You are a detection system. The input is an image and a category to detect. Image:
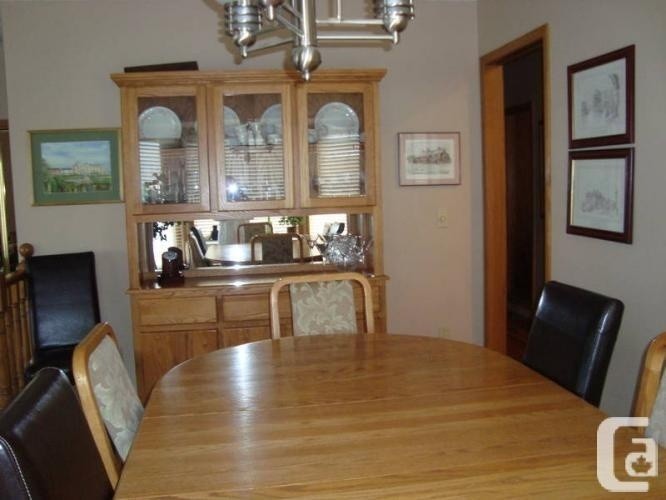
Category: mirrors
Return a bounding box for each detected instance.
[136,213,374,280]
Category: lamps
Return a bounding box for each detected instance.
[221,1,416,83]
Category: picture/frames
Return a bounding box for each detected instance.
[565,147,634,244]
[26,128,125,207]
[567,44,635,149]
[398,131,461,186]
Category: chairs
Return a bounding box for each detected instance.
[0,366,115,500]
[629,331,666,436]
[72,322,145,491]
[189,223,345,266]
[269,272,375,339]
[24,252,100,385]
[522,280,625,408]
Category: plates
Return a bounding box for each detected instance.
[139,101,360,205]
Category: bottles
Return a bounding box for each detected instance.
[211,225,219,240]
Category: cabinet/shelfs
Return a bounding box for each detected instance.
[110,68,389,409]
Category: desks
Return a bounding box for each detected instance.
[111,333,666,500]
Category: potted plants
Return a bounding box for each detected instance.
[279,216,305,235]
